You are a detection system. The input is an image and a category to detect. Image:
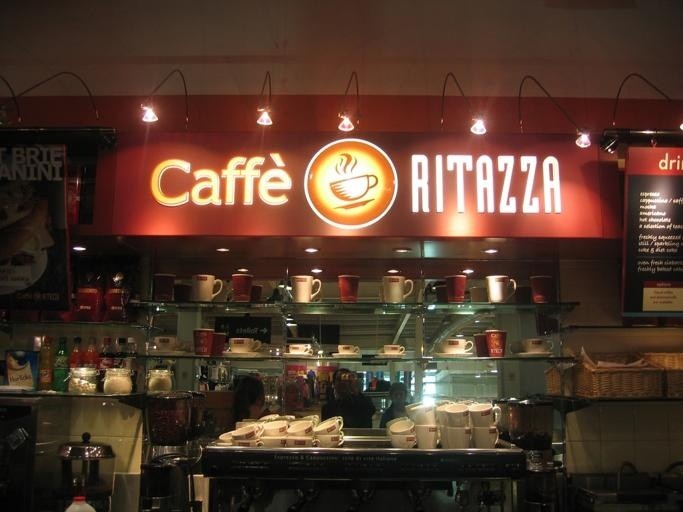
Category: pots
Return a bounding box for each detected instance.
[493,392,555,450]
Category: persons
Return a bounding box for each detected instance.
[322,368,374,434]
[222,371,269,431]
[378,383,419,431]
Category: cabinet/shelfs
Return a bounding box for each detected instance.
[0,302,578,476]
[204,437,524,512]
[562,327,683,512]
[1,390,143,511]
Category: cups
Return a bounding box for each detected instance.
[484,329,506,357]
[383,345,404,354]
[337,274,359,302]
[444,275,467,303]
[286,420,316,436]
[391,434,417,449]
[231,274,253,302]
[409,402,439,424]
[522,338,553,352]
[216,442,233,446]
[258,436,286,447]
[213,332,226,355]
[232,424,264,439]
[386,399,480,442]
[153,337,176,351]
[288,344,311,353]
[445,426,473,449]
[257,413,342,428]
[389,420,416,435]
[485,275,516,303]
[529,275,556,303]
[439,338,474,352]
[382,275,413,304]
[228,338,260,353]
[290,275,321,303]
[337,345,359,354]
[472,333,488,357]
[285,436,320,448]
[261,420,288,436]
[192,328,214,355]
[230,438,264,447]
[190,275,223,301]
[439,424,449,448]
[312,418,343,435]
[436,404,451,426]
[445,403,471,427]
[314,434,344,448]
[218,429,236,443]
[414,425,440,448]
[471,426,499,448]
[468,403,502,427]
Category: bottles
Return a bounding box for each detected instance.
[54,338,69,393]
[126,338,137,394]
[65,497,95,512]
[115,336,126,369]
[83,337,98,369]
[70,337,82,372]
[97,337,113,393]
[38,336,53,391]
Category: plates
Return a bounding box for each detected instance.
[331,353,362,357]
[375,353,406,359]
[429,352,474,358]
[282,352,312,358]
[518,351,554,357]
[222,351,260,358]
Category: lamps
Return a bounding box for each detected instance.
[518,75,591,148]
[140,67,191,131]
[256,71,275,128]
[3,71,101,126]
[337,71,363,132]
[440,72,486,135]
[612,73,683,131]
[599,128,629,155]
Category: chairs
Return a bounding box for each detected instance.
[138,393,204,512]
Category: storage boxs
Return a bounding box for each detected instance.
[644,281,683,311]
[638,352,681,397]
[574,354,666,398]
[544,364,573,395]
